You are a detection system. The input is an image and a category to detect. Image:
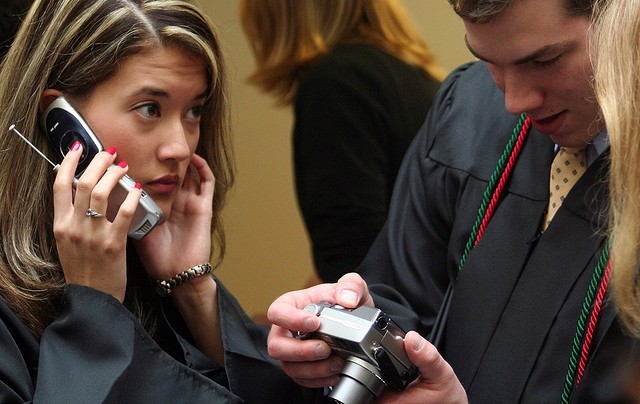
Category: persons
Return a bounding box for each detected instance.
[236,0,452,285]
[266,1,640,404]
[583,0,640,341]
[0,0,333,404]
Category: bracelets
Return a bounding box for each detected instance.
[153,261,213,298]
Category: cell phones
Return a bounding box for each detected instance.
[7,96,164,242]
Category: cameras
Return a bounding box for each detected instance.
[297,300,422,404]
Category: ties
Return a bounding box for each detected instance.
[543,146,588,234]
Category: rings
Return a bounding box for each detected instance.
[85,207,106,218]
